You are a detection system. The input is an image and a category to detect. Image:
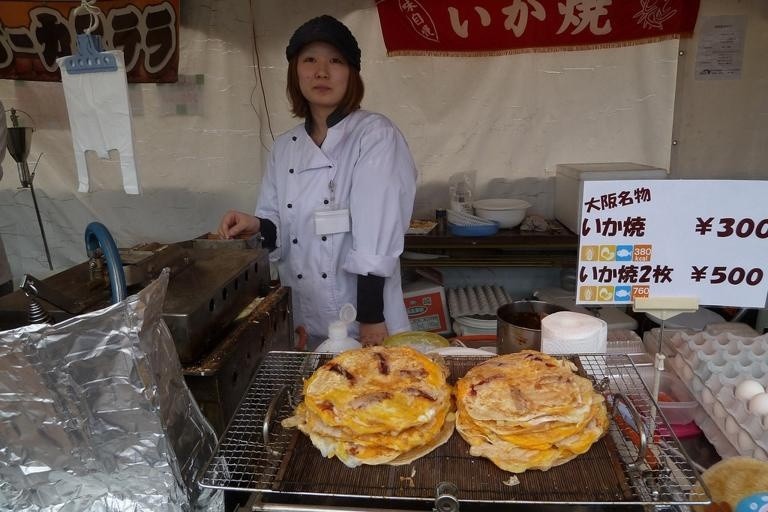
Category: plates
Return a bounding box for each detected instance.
[445,208,501,236]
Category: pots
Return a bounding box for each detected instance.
[494,302,572,357]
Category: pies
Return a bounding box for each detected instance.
[296,345,455,473]
[453,349,609,473]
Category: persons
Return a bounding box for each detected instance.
[217,14,419,347]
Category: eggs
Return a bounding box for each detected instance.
[674,353,768,462]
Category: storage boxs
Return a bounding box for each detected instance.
[542,297,638,330]
[632,371,699,425]
[553,163,668,235]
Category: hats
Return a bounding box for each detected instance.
[286,15,361,67]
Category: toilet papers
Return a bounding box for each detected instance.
[541,309,607,380]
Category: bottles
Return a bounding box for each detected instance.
[312,304,361,354]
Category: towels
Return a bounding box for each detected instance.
[520,215,549,232]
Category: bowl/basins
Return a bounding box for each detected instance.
[472,198,532,229]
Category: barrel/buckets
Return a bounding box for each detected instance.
[450,312,497,354]
